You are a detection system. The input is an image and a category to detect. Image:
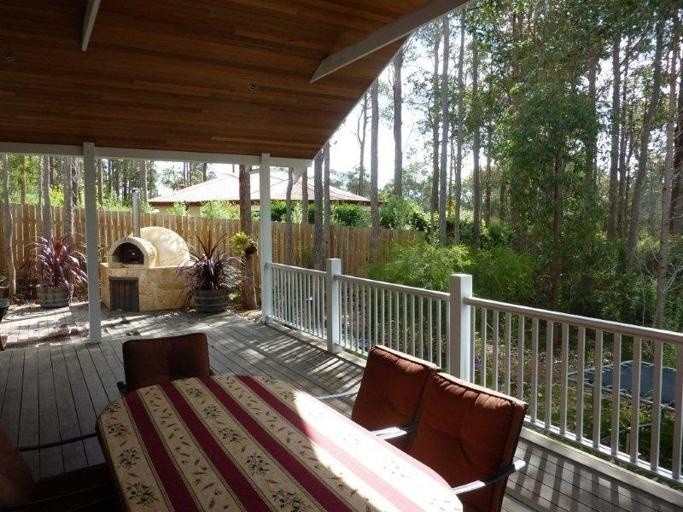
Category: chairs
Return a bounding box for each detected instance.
[377,371,529,511]
[1,419,128,512]
[315,344,442,453]
[116,333,216,395]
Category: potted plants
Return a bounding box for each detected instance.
[21,232,88,308]
[175,234,243,313]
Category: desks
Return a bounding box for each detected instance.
[94,374,462,512]
